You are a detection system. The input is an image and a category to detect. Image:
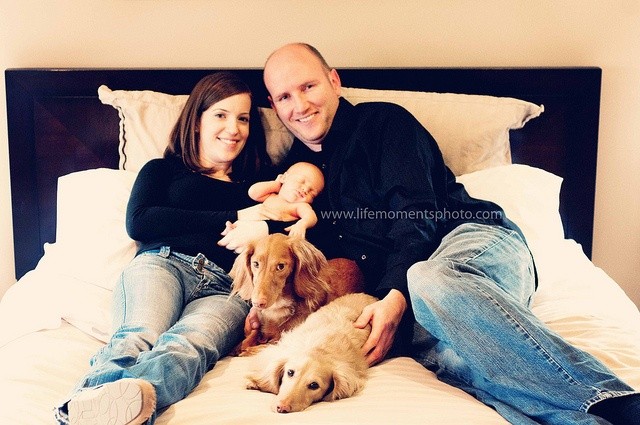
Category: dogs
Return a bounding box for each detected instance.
[227,233,365,356]
[242,293,380,414]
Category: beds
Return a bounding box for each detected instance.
[3,65,640,424]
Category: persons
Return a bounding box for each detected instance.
[226,162,325,239]
[51,70,340,425]
[244,43,639,425]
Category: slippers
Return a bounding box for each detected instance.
[68,379,158,425]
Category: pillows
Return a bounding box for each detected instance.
[344,86,546,174]
[97,83,294,171]
